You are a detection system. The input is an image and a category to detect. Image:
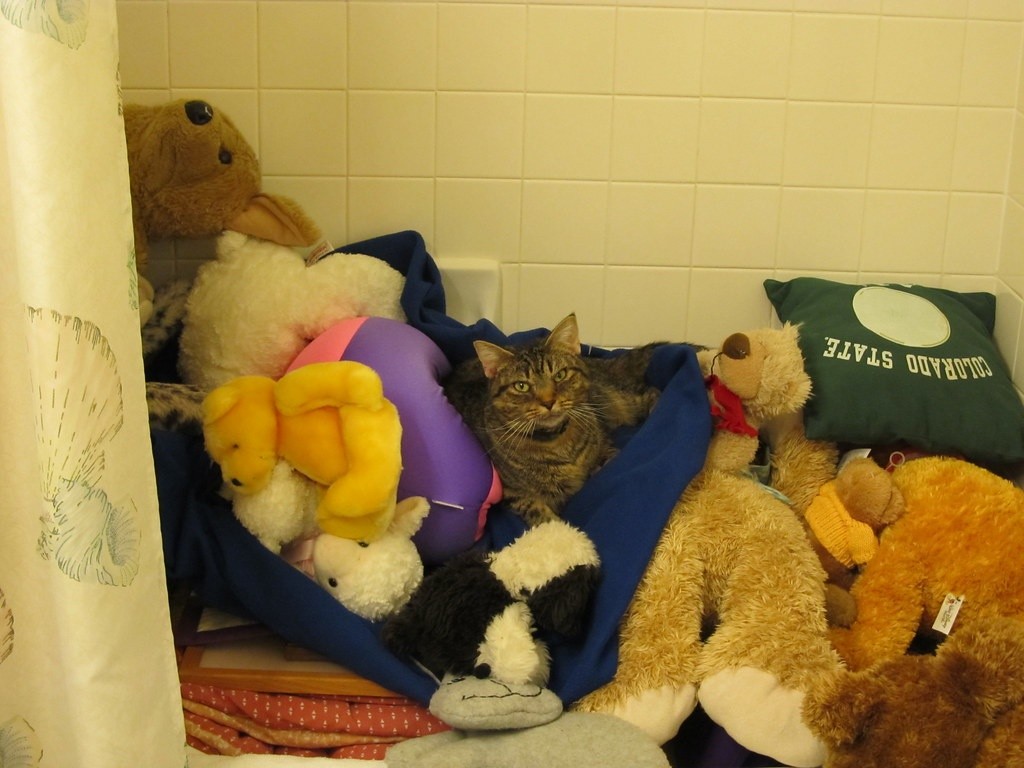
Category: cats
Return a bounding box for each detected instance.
[450,312,710,526]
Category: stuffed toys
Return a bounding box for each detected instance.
[121,96,1024,768]
